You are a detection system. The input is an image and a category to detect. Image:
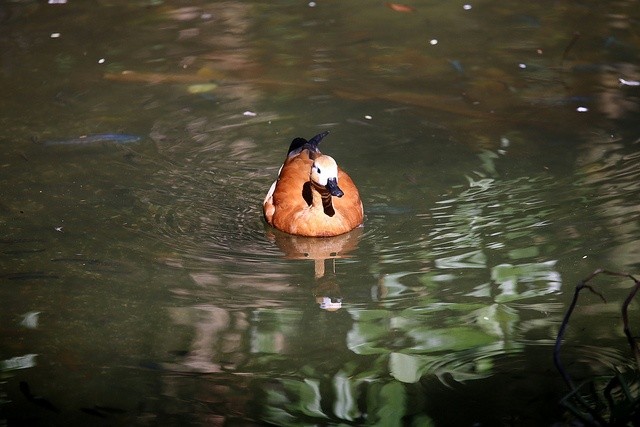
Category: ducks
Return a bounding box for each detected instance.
[263,129,365,237]
